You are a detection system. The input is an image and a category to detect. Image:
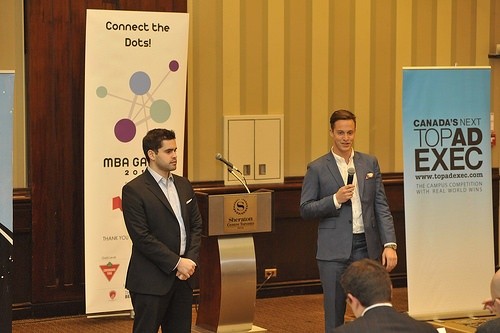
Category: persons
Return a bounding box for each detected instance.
[475,268,500,333]
[299,109,397,333]
[334,258,439,333]
[122,129,202,333]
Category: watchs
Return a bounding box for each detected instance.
[384,244,397,250]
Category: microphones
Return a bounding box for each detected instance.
[347,167,356,184]
[216,153,241,173]
